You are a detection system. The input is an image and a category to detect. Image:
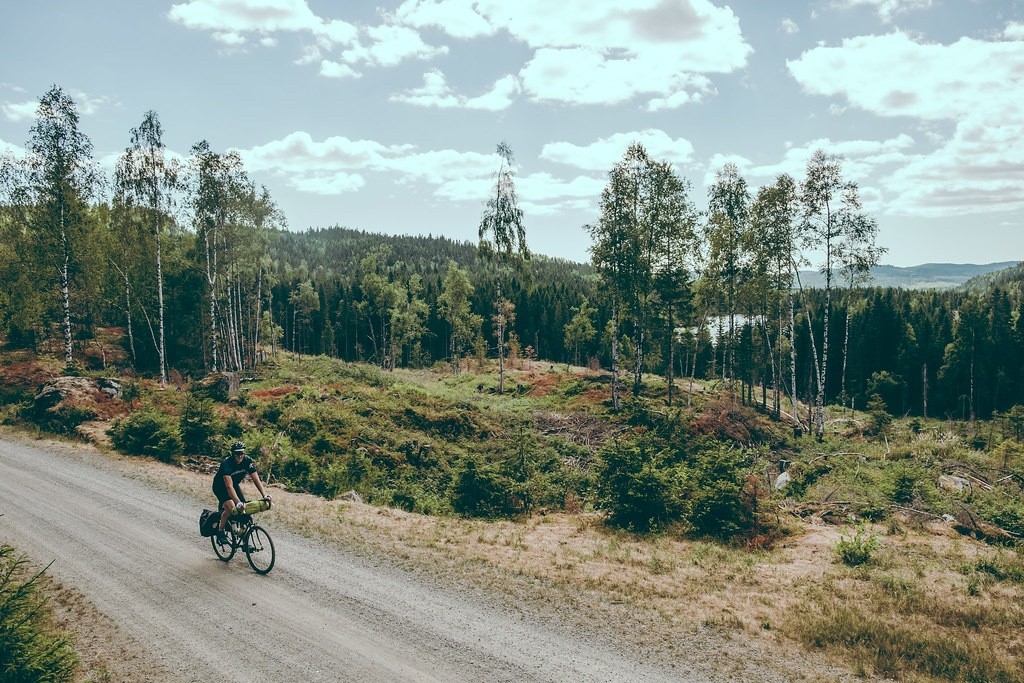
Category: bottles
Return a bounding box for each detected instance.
[239,524,246,537]
[231,522,237,533]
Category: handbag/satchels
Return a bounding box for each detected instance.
[200,508,221,538]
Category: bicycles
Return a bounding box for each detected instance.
[210,495,275,574]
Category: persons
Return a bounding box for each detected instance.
[212,443,272,552]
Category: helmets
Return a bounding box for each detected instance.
[231,441,246,452]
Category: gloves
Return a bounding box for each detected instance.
[235,501,246,513]
[264,495,272,500]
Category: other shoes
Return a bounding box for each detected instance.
[242,545,253,552]
[217,531,228,543]
